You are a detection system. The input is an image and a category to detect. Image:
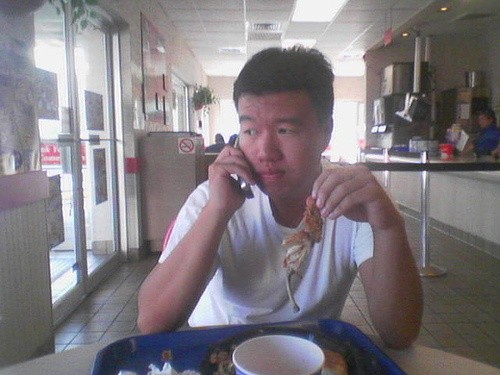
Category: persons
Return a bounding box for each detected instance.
[468,107,499,158]
[138,43,424,349]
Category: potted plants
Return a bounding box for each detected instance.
[195,82,219,111]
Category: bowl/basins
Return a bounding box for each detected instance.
[232,335,325,375]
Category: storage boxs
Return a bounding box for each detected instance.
[372,96,406,127]
[371,121,428,153]
[381,60,429,95]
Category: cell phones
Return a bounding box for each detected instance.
[233,135,255,199]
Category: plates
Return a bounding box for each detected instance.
[203,326,386,375]
[92,320,404,374]
